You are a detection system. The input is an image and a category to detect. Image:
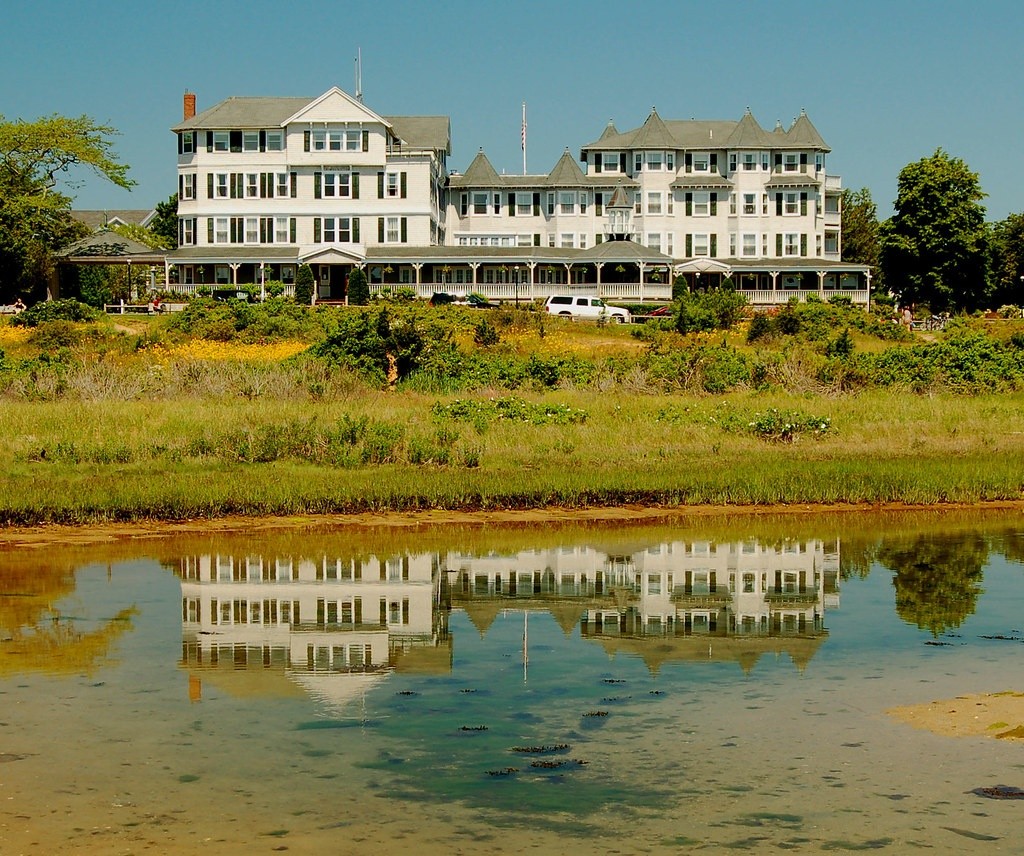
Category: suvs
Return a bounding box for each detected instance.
[544,295,631,324]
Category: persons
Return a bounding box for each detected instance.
[13,298,27,315]
[904,306,913,331]
[152,296,163,315]
